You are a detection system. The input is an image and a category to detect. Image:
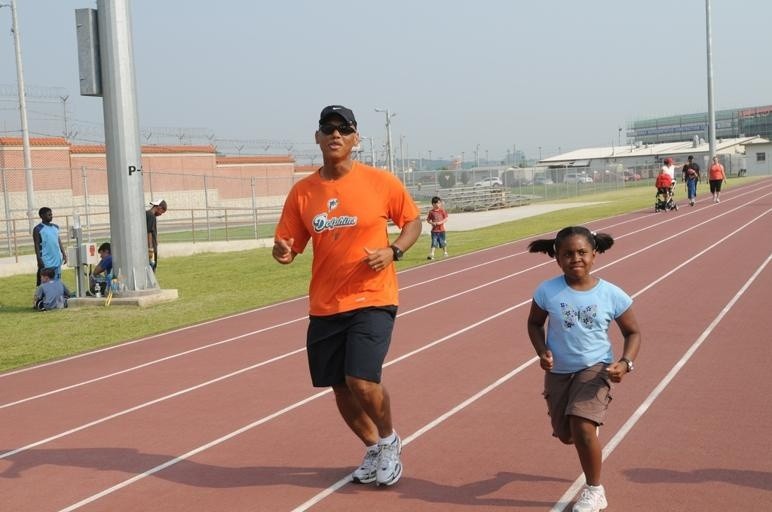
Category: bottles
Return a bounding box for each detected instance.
[95,282,101,297]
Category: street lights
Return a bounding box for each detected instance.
[360,135,375,168]
[426,144,562,166]
[618,127,623,145]
[375,108,399,176]
[399,134,406,160]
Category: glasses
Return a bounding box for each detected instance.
[322,125,354,136]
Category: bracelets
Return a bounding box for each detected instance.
[619,357,633,373]
[390,244,403,261]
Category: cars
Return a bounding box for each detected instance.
[475,177,503,190]
[563,173,593,185]
[587,170,640,181]
[527,176,553,186]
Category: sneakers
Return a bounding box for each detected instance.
[353,434,402,487]
[572,484,608,512]
[427,255,435,259]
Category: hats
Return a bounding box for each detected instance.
[150,200,167,211]
[320,106,357,132]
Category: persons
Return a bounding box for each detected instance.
[85,243,113,296]
[686,168,697,207]
[33,207,69,286]
[32,268,70,311]
[706,156,727,204]
[526,225,643,511]
[146,199,168,273]
[273,105,423,488]
[426,196,449,260]
[658,156,675,204]
[681,154,700,196]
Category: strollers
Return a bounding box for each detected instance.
[655,174,679,214]
